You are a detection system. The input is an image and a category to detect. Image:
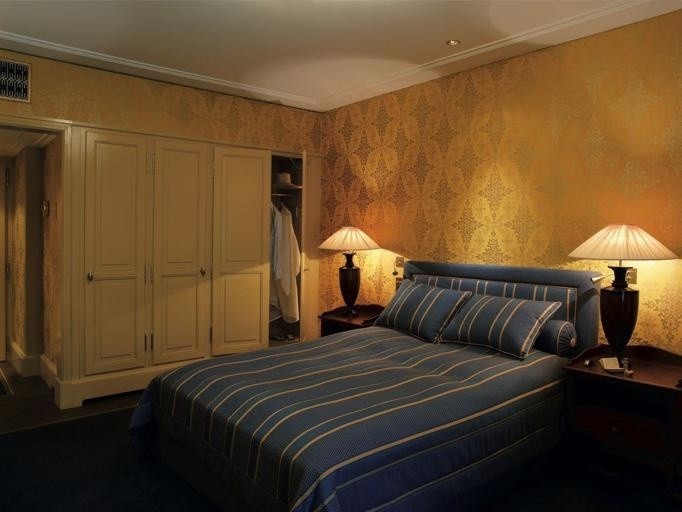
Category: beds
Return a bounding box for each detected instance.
[128,260,602,509]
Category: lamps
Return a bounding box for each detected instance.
[317,226,380,319]
[568,224,680,377]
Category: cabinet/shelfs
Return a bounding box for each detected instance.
[60,124,322,411]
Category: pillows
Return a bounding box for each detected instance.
[373,278,578,362]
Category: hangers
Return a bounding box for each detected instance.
[271,196,291,217]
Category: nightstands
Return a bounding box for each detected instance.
[318,303,386,337]
[563,343,680,511]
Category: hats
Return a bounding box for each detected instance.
[272,172,302,189]
